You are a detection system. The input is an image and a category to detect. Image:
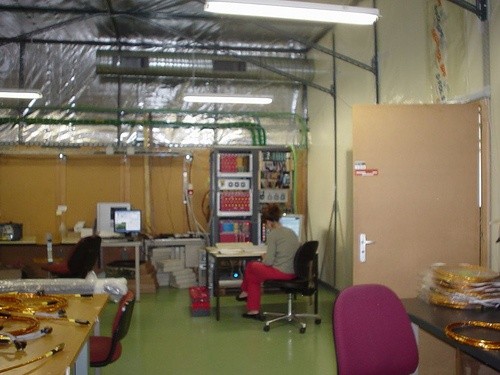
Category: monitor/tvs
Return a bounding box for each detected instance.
[114,209,142,239]
[280,214,304,241]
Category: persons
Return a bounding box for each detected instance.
[236,205,301,320]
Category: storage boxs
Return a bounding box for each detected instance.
[107,246,200,293]
[0,268,22,281]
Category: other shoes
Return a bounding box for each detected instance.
[236,294,247,301]
[241,312,262,320]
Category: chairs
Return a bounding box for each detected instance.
[41,234,102,279]
[332,284,419,375]
[88,290,135,368]
[260,240,322,333]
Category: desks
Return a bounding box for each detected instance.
[400,297,500,375]
[0,275,128,375]
[203,247,318,320]
[0,237,206,278]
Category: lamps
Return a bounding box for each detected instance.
[183,80,273,105]
[203,0,380,26]
[0,88,44,99]
